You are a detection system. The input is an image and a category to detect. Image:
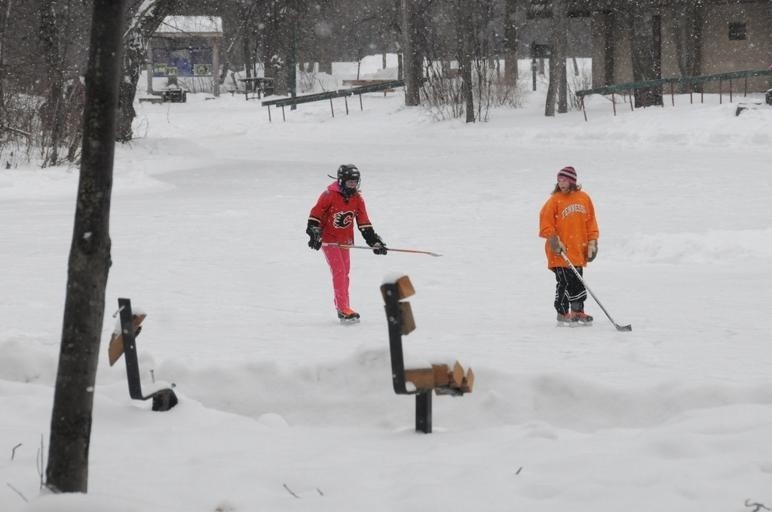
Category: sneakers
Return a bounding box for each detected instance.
[556,311,593,323]
[337,312,361,319]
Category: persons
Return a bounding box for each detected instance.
[306,164,388,320]
[538,167,599,322]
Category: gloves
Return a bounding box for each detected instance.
[366,233,387,255]
[305,225,322,251]
[549,235,566,256]
[586,239,598,262]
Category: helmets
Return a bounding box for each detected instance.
[338,164,361,193]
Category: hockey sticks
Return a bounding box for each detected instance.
[308,241,442,258]
[559,249,632,331]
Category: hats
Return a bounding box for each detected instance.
[557,166,577,184]
